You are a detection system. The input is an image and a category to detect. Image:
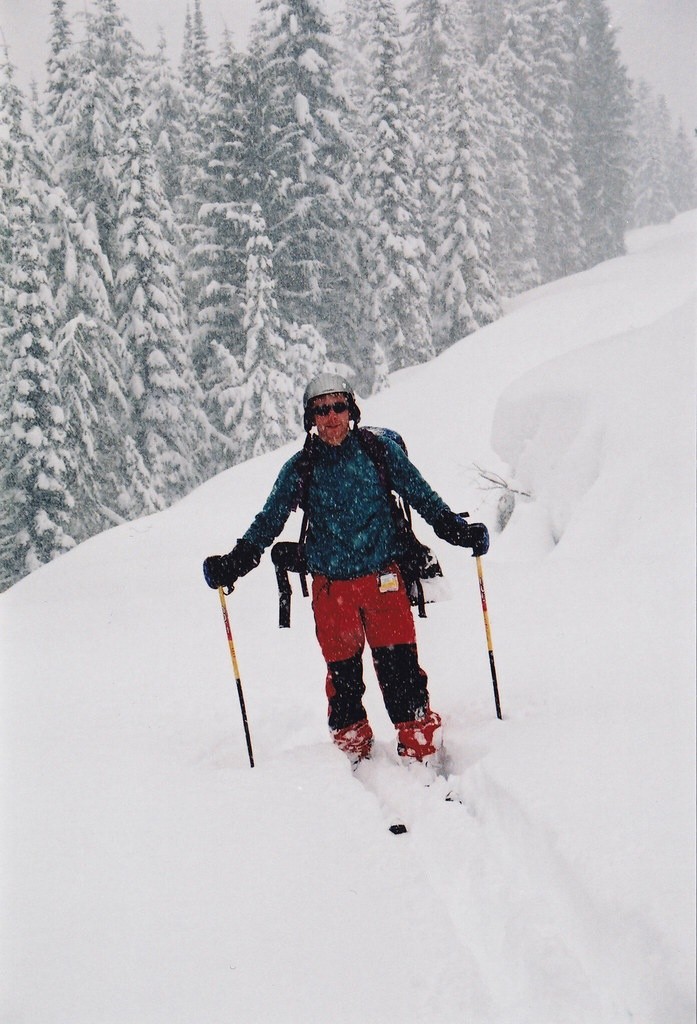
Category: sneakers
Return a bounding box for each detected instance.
[395,712,441,766]
[328,719,374,773]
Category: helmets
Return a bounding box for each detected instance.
[303,372,355,411]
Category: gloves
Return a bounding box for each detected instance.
[433,510,490,557]
[203,539,264,595]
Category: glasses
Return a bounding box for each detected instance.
[311,401,349,416]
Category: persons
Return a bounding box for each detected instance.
[202,374,490,777]
